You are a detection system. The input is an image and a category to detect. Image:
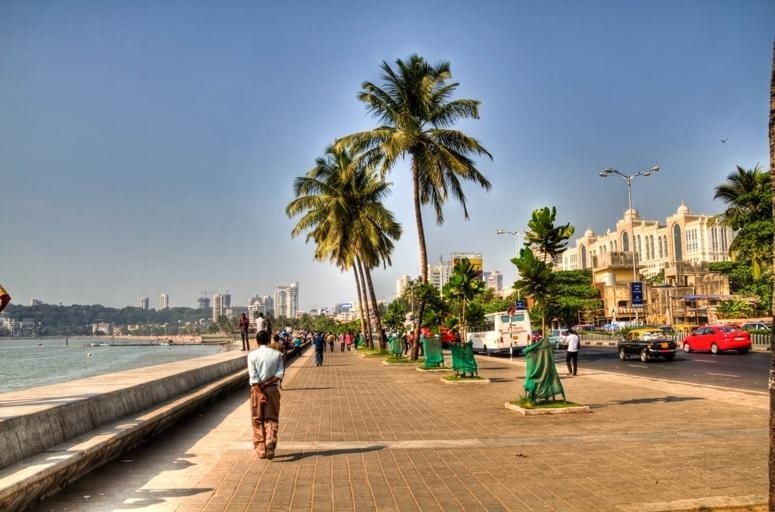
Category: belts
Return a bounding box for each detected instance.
[267,384,278,386]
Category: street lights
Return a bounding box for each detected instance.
[598,166,660,329]
[496,228,527,310]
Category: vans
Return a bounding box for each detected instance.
[548,328,570,349]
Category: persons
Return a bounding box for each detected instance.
[247,330,286,460]
[266,317,371,390]
[239,312,250,351]
[254,312,265,333]
[562,328,580,376]
[382,329,425,358]
[531,327,542,342]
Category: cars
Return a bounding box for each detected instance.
[657,326,676,333]
[573,324,596,332]
[532,329,543,343]
[741,322,770,331]
[618,329,677,363]
[682,324,752,356]
[600,323,620,334]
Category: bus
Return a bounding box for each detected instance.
[465,309,533,357]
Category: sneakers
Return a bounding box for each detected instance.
[266,441,275,460]
